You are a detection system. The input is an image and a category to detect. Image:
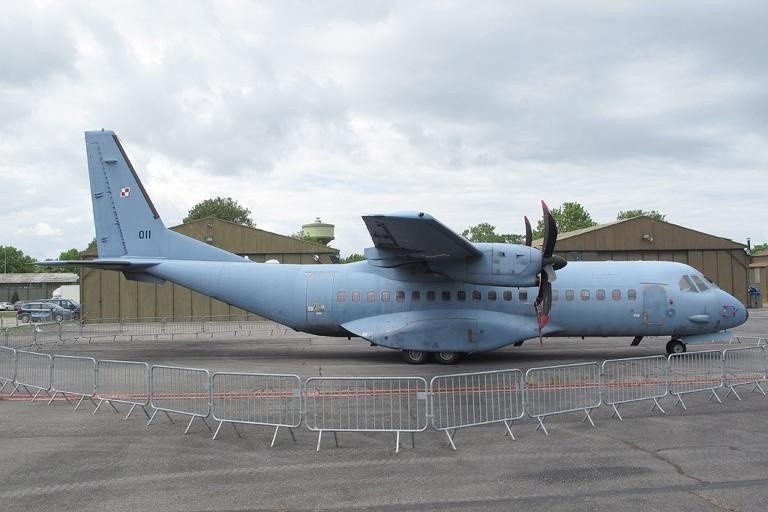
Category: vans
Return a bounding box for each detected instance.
[44,299,79,315]
[16,302,74,323]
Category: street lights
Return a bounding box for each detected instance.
[2,243,7,274]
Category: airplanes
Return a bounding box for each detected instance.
[25,129,749,365]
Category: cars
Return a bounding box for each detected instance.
[0,302,14,311]
[14,300,23,310]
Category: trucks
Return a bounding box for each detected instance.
[49,285,80,306]
